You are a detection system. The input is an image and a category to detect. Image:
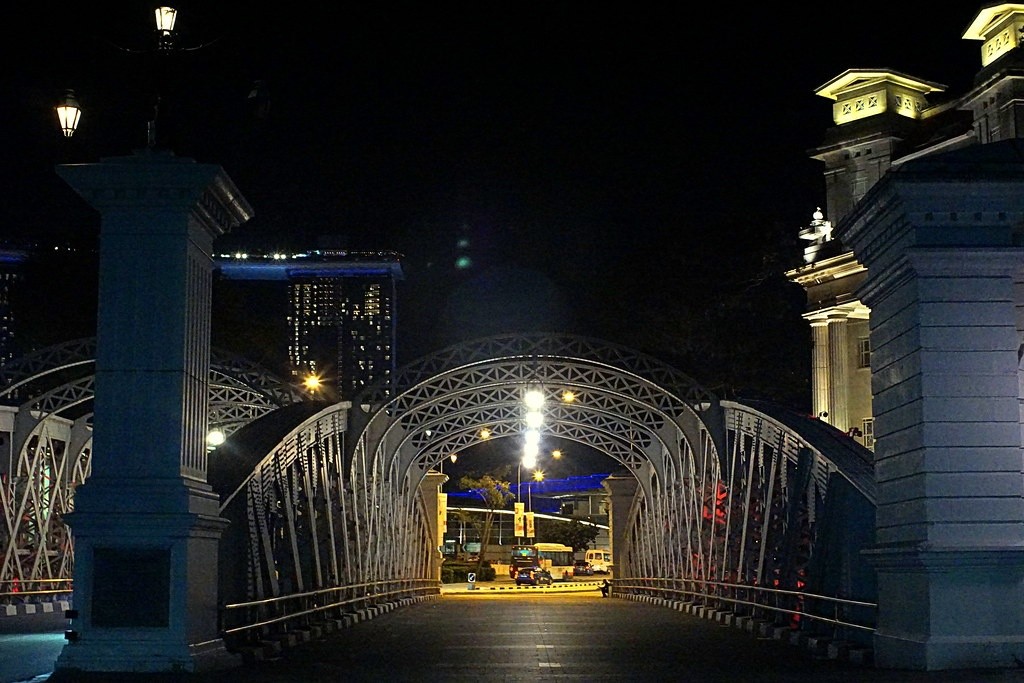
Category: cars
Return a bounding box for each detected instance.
[574,562,595,575]
[515,567,558,584]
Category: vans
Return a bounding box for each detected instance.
[586,549,612,574]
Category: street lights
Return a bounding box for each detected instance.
[528,470,545,544]
[517,450,561,544]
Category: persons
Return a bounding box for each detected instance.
[599,579,610,598]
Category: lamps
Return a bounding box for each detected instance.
[846,427,862,438]
[49,89,82,154]
[819,411,829,418]
[153,0,178,38]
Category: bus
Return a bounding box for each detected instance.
[509,543,574,581]
